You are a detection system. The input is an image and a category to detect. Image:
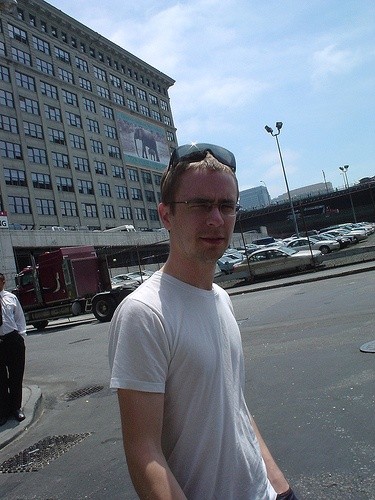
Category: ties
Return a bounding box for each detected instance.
[0,300,3,327]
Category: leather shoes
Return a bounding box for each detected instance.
[0,412,8,425]
[14,408,25,422]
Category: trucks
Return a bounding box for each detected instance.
[11,245,112,330]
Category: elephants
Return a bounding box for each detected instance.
[133,126,161,162]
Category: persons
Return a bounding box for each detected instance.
[109,143,296,500]
[0,272,27,426]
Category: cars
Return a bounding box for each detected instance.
[230,246,324,282]
[286,236,341,254]
[215,235,282,276]
[91,270,155,323]
[283,222,375,250]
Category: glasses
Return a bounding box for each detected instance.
[161,143,236,189]
[164,200,247,216]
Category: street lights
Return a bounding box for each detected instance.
[265,121,299,237]
[338,164,357,223]
